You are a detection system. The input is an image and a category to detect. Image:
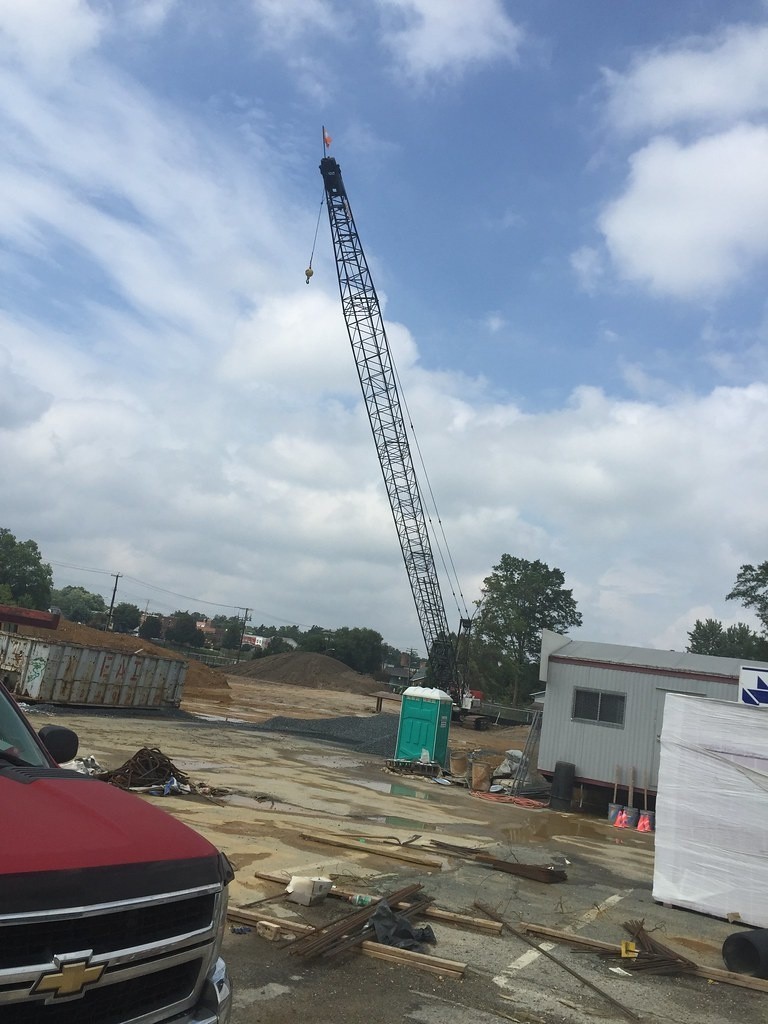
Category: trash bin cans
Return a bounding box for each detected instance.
[394,693,454,769]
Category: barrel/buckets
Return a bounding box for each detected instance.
[450,751,468,775]
[608,803,623,824]
[623,806,638,826]
[472,760,490,791]
[640,810,655,828]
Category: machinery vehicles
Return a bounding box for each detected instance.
[305,126,491,731]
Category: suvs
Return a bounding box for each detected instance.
[0,678,233,1024]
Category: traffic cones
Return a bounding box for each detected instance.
[635,814,644,831]
[642,814,651,831]
[619,811,629,828]
[614,809,622,826]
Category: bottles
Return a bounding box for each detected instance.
[349,894,371,906]
